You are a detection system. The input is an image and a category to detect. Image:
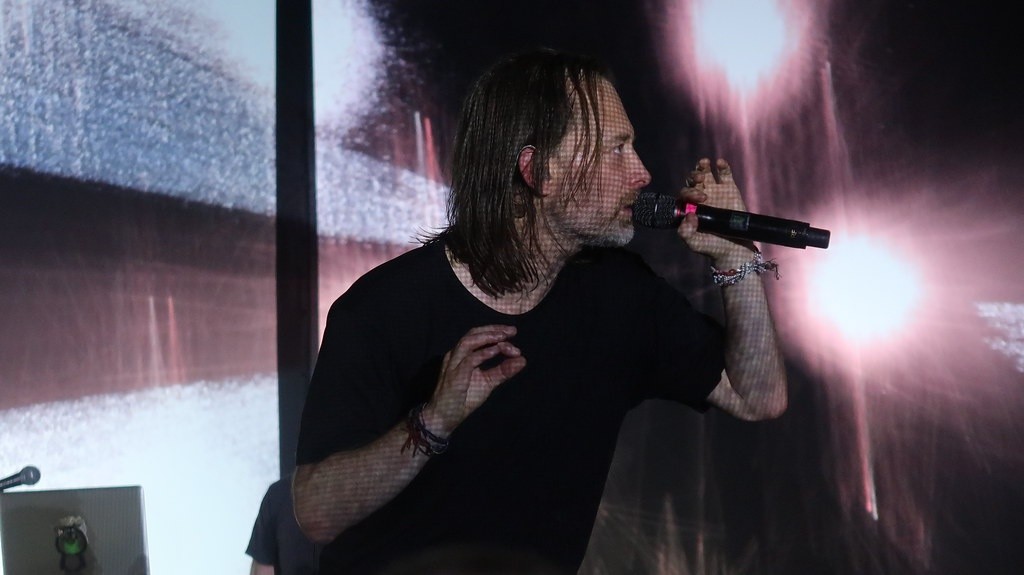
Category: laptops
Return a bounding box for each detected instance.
[0,486,150,575]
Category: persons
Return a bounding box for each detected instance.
[246,468,325,575]
[293,47,790,575]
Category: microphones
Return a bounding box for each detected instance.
[632,192,831,250]
[0,466,41,489]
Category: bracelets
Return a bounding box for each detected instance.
[710,250,780,287]
[402,404,449,459]
